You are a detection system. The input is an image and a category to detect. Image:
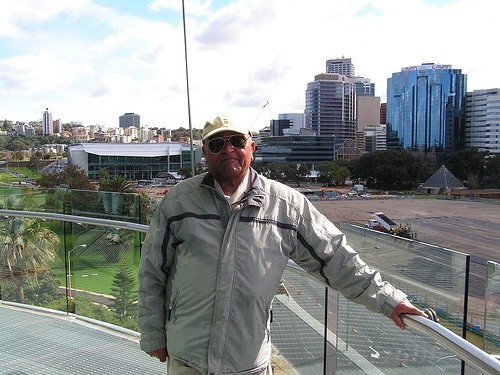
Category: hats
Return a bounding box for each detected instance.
[201,113,253,141]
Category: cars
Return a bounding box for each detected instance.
[306,185,400,201]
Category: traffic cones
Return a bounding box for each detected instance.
[315,297,320,305]
[398,356,404,367]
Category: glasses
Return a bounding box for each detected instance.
[204,135,250,153]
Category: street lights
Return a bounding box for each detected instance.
[67,243,87,301]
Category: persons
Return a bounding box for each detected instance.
[138,113,429,375]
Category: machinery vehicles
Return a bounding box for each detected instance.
[364,211,397,235]
